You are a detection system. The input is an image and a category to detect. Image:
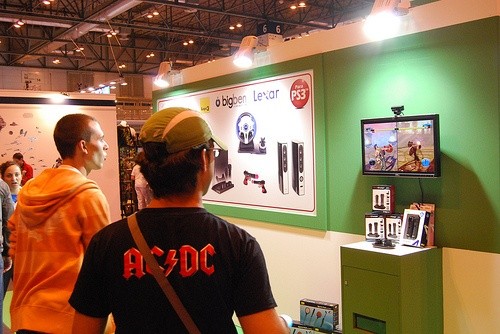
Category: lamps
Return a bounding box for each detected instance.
[152,62,171,89]
[369,0,411,16]
[232,36,258,68]
[78,80,127,94]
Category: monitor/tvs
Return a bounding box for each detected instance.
[361,113,441,179]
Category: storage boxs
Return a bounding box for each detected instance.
[289,298,341,334]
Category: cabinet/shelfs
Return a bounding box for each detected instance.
[341,241,443,334]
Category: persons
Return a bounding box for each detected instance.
[131,152,151,210]
[0,160,24,211]
[67,107,292,334]
[6,113,116,334]
[12,153,33,186]
[0,113,14,333]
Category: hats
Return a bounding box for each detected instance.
[139,108,227,167]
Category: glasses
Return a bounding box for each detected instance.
[208,148,220,158]
